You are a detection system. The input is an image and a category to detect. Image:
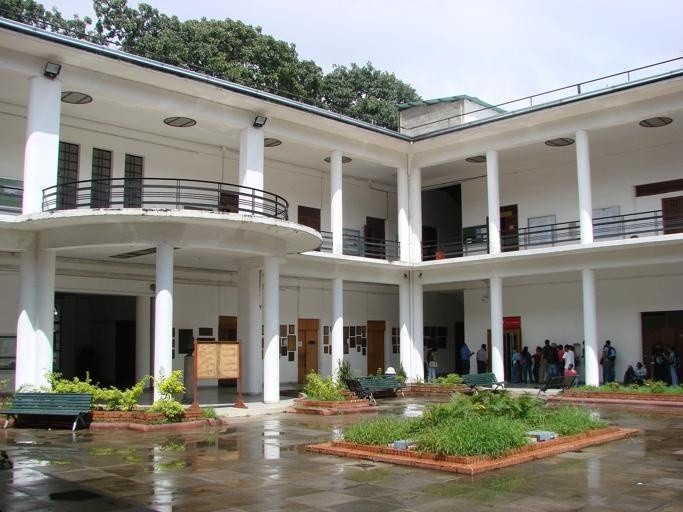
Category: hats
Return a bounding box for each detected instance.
[385,367,396,374]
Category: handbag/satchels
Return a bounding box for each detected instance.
[429,360,438,367]
[607,347,616,360]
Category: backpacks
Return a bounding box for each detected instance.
[672,356,681,368]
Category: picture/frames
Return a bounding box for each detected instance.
[280,325,297,360]
[391,327,448,353]
[324,326,366,355]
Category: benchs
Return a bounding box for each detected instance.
[0,392,93,432]
[346,375,405,405]
[534,376,577,397]
[462,372,504,395]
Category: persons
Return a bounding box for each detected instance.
[601,340,616,384]
[512,339,578,387]
[461,344,475,374]
[624,340,683,389]
[581,341,584,358]
[424,343,439,384]
[477,344,488,373]
